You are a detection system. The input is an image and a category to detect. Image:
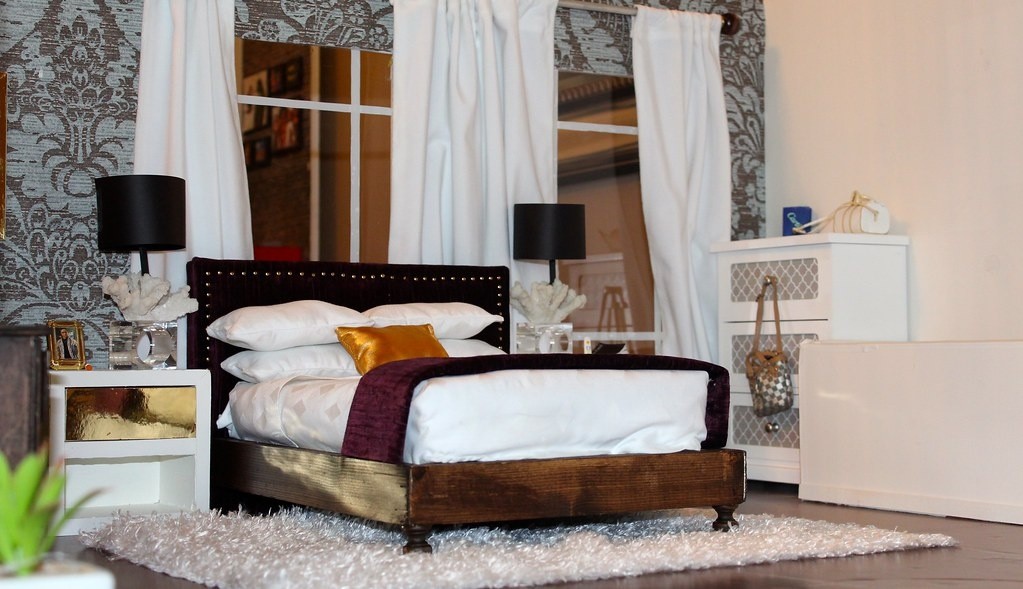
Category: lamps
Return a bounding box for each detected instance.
[512,203,585,284]
[95,175,186,275]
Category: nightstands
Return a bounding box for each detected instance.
[47,369,211,536]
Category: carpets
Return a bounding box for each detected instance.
[76,505,955,589]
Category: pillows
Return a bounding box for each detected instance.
[206,299,375,353]
[440,338,506,358]
[221,343,362,384]
[362,303,504,339]
[336,323,450,377]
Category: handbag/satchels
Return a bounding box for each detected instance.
[746,349,794,417]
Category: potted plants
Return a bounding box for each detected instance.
[0,441,115,588]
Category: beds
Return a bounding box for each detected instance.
[186,257,747,555]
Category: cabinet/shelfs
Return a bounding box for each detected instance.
[710,232,911,483]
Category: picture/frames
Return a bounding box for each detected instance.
[49,320,86,370]
[242,56,304,170]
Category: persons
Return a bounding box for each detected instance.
[56,329,79,359]
